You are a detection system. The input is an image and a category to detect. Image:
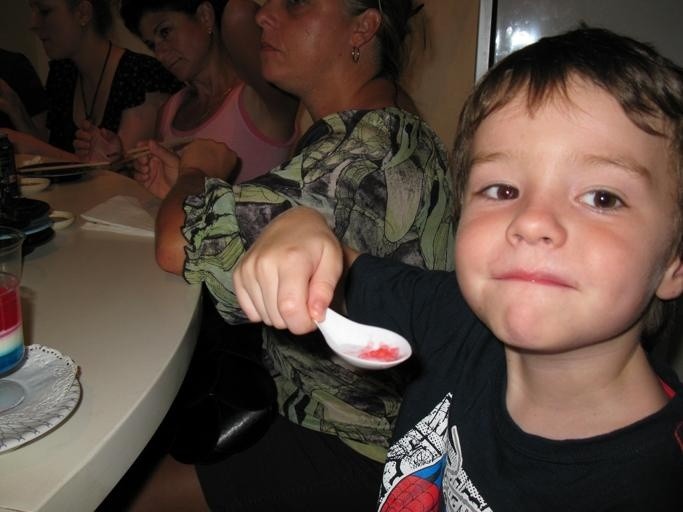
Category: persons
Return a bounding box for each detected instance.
[120,2,459,511]
[72,0,304,189]
[231,22,682,511]
[0,48,48,145]
[0,0,186,164]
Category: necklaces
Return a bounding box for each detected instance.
[175,75,244,131]
[71,37,115,125]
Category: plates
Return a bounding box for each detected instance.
[48,211,73,230]
[0,345,82,454]
[18,177,49,194]
[19,162,92,183]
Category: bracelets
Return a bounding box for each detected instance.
[174,166,212,186]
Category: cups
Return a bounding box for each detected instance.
[0,222,29,376]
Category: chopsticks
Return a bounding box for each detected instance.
[126,138,189,162]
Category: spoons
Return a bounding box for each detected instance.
[312,296,413,376]
